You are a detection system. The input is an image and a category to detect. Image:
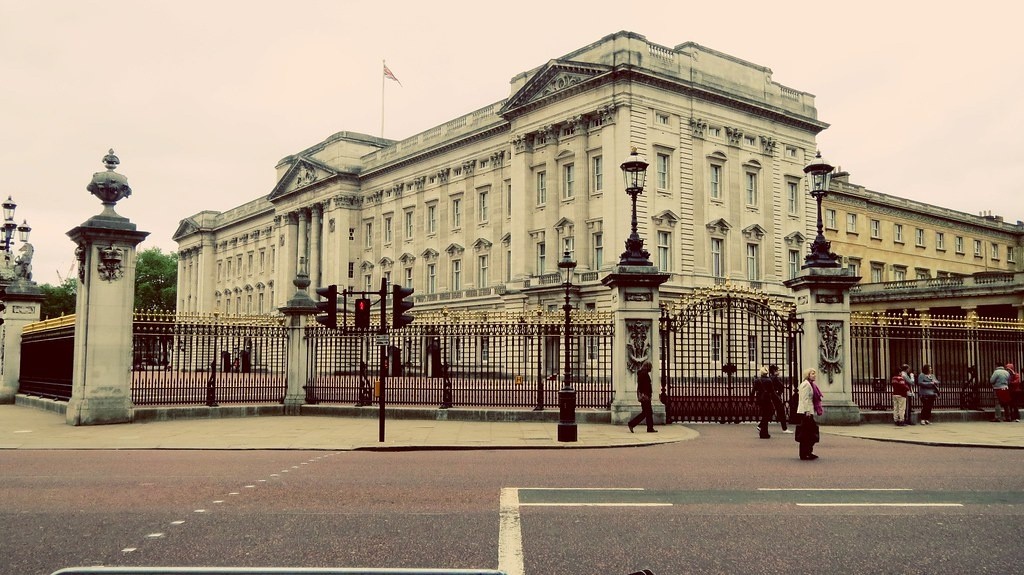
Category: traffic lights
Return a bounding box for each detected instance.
[393,284,415,330]
[315,284,338,329]
[354,299,370,330]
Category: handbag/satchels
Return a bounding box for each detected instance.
[795,416,820,442]
[638,392,650,402]
[934,384,941,396]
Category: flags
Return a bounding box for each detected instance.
[383,65,402,87]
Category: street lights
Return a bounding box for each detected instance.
[802,148,844,267]
[0,195,17,253]
[617,145,659,266]
[557,246,578,441]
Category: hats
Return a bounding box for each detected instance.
[760,367,769,376]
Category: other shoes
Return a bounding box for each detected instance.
[783,429,794,433]
[628,421,635,433]
[921,419,930,425]
[906,420,916,425]
[1013,418,1020,421]
[760,435,770,439]
[800,454,818,460]
[990,417,1000,422]
[647,428,658,432]
[757,426,761,432]
[895,421,906,426]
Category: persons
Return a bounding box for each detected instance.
[1005,364,1021,422]
[891,367,910,427]
[918,365,939,426]
[900,364,916,425]
[796,369,819,461]
[990,363,1011,422]
[749,367,778,439]
[628,360,658,433]
[757,365,793,434]
